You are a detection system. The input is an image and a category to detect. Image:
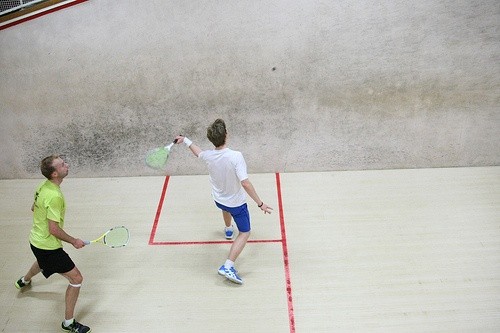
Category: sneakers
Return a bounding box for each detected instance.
[15,276,31,289]
[218,265,243,284]
[61,318,94,333]
[224,224,233,238]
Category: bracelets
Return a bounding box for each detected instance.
[257,202,263,207]
[183,137,192,148]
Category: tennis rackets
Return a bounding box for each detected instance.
[144,135,182,169]
[82,224,130,249]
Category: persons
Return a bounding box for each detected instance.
[174,119,273,285]
[15,155,92,333]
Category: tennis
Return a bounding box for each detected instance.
[271,65,277,72]
